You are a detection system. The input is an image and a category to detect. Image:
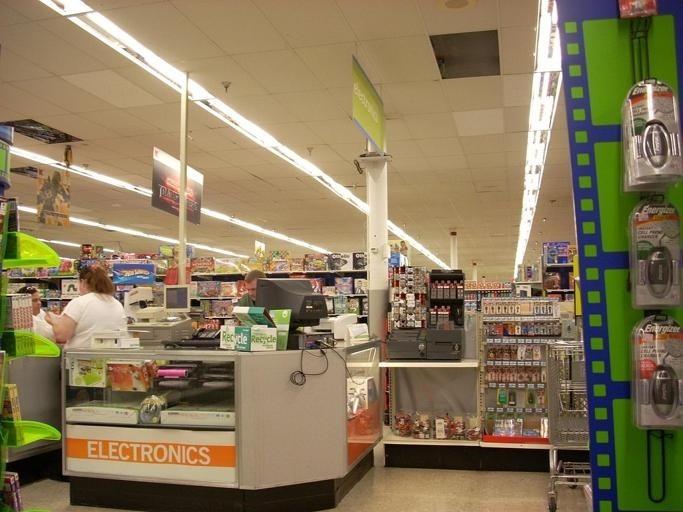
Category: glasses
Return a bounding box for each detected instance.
[24,286,37,293]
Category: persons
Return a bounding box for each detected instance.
[235,268,267,307]
[37,169,69,226]
[43,264,128,349]
[15,286,57,343]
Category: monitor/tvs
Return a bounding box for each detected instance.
[256,279,328,332]
[163,285,191,312]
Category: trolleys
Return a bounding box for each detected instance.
[539,341,595,512]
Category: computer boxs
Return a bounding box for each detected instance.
[288,332,334,350]
